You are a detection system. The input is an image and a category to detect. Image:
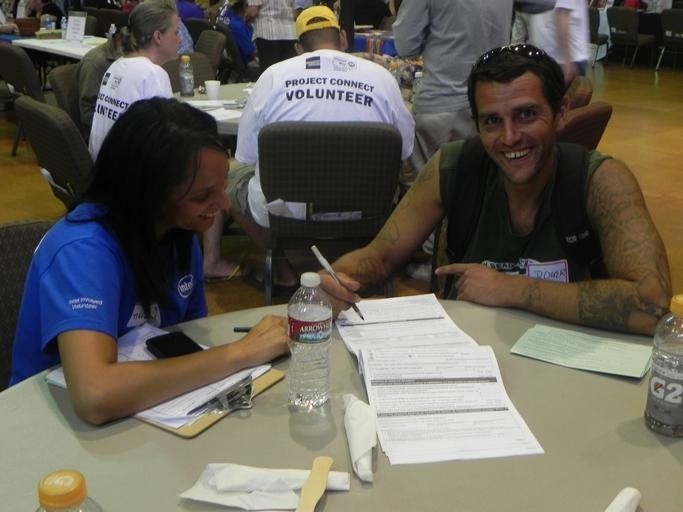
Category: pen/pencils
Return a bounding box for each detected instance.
[234,327,252,336]
[311,245,367,320]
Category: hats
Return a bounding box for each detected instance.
[294,5,340,38]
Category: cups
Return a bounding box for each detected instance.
[204,79,221,101]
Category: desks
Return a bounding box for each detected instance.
[0,293,680,510]
[170,79,422,139]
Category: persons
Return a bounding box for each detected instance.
[11,96,301,425]
[168,1,297,75]
[294,44,671,341]
[211,5,420,297]
[0,1,137,143]
[389,0,559,284]
[555,0,591,85]
[80,0,255,281]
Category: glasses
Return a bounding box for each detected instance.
[474,43,545,69]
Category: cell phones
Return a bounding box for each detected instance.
[146,331,203,359]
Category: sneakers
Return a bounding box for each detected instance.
[256,263,299,295]
[407,260,431,282]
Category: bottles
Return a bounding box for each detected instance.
[44,14,49,30]
[178,56,195,98]
[108,24,116,34]
[32,470,106,512]
[287,271,332,406]
[60,17,67,40]
[412,72,425,100]
[642,292,682,437]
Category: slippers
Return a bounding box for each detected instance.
[205,264,248,283]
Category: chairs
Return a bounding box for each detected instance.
[586,6,681,72]
[553,99,612,153]
[12,95,97,209]
[8,2,402,95]
[47,62,81,139]
[561,75,592,112]
[250,115,408,310]
[0,42,48,156]
